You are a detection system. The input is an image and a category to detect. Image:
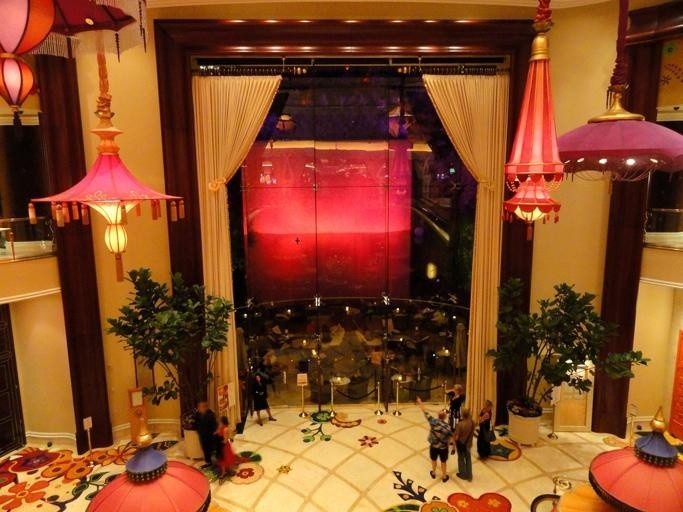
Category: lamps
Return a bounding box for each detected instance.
[500,0,566,242]
[0,1,148,144]
[386,102,414,139]
[23,19,189,285]
[555,2,682,185]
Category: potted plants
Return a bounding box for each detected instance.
[102,268,235,460]
[481,282,653,446]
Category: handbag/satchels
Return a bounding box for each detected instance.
[489,430,496,442]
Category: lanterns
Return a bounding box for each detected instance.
[2,1,54,143]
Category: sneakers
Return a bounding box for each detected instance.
[430,471,435,478]
[443,475,449,482]
[456,473,466,480]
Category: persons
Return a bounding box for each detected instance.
[214,415,238,479]
[251,372,277,426]
[192,395,224,468]
[416,384,492,482]
